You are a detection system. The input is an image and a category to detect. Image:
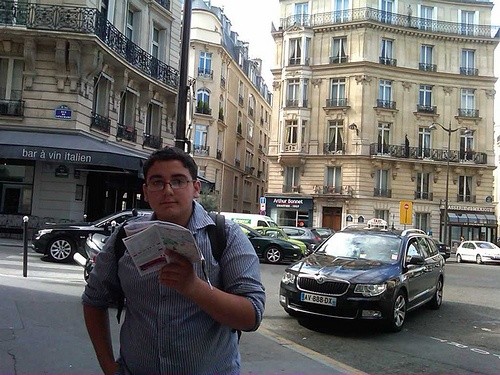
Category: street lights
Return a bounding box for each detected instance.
[428,121,472,244]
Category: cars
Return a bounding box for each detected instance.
[455,240,500,265]
[278,219,445,333]
[30,210,155,264]
[430,237,451,262]
[238,223,303,264]
[247,223,336,258]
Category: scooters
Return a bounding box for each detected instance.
[83,207,138,284]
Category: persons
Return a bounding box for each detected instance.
[460,236,465,242]
[297,221,308,227]
[82,146,266,375]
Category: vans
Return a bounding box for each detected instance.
[211,210,280,235]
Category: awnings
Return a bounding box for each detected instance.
[446,212,497,224]
[0,130,149,170]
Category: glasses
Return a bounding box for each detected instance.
[148,179,197,192]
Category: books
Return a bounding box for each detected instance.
[122,221,203,277]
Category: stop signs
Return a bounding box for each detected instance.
[404,204,409,210]
